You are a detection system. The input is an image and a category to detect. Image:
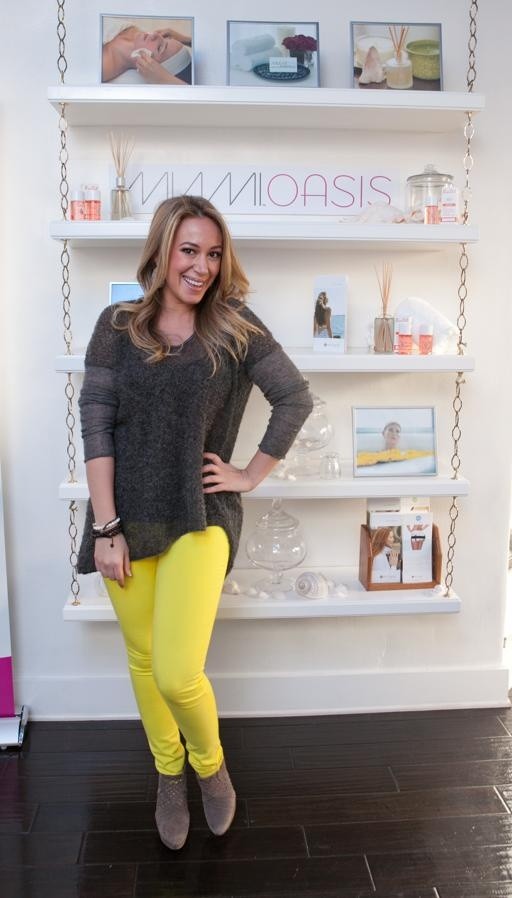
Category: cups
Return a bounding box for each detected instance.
[318,451,343,480]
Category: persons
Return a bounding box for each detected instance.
[372,527,400,583]
[358,421,434,466]
[102,16,190,83]
[75,195,312,850]
[136,26,192,85]
[314,290,333,338]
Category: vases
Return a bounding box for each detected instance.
[290,50,313,67]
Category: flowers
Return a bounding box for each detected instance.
[282,33,317,53]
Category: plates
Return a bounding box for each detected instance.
[404,39,441,57]
[252,62,312,81]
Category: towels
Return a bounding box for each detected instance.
[230,34,283,72]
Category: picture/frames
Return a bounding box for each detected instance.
[349,21,444,92]
[108,281,142,307]
[226,19,321,88]
[350,404,440,477]
[99,13,196,89]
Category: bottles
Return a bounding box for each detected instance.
[404,162,455,225]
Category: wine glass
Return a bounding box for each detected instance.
[245,499,309,595]
[276,381,334,480]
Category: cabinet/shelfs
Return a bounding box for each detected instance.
[43,80,487,620]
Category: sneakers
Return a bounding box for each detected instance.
[195,759,238,837]
[154,764,190,851]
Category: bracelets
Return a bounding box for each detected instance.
[89,516,124,549]
